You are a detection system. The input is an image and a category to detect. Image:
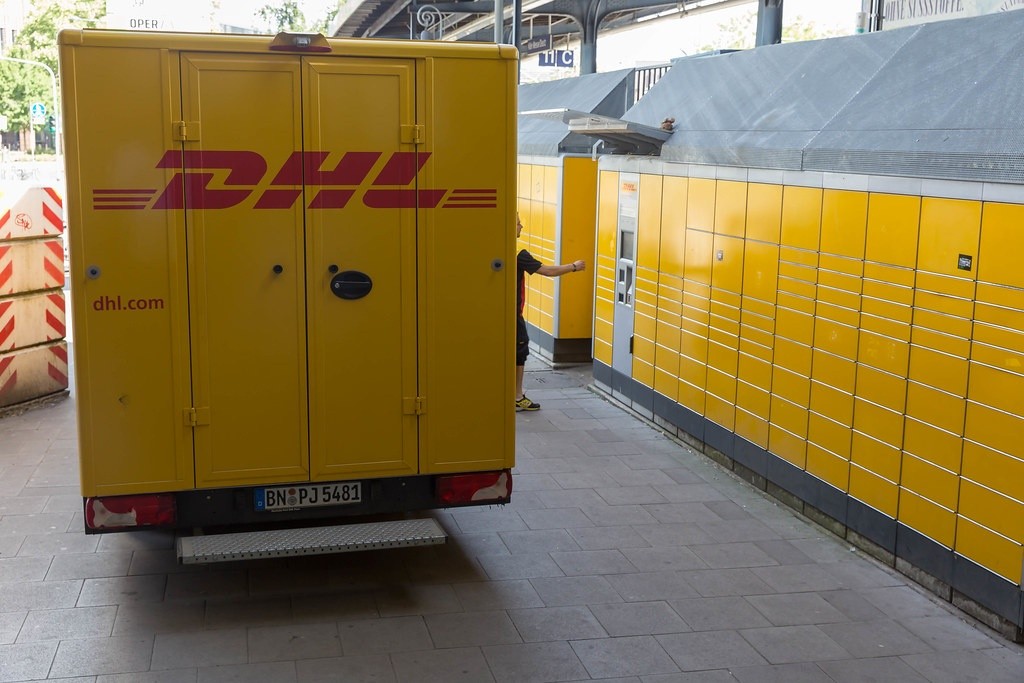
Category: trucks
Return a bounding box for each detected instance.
[53,26,517,569]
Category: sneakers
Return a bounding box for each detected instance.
[516,394,540,412]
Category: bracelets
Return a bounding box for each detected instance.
[573,264,576,272]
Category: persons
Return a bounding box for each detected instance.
[516,211,586,412]
